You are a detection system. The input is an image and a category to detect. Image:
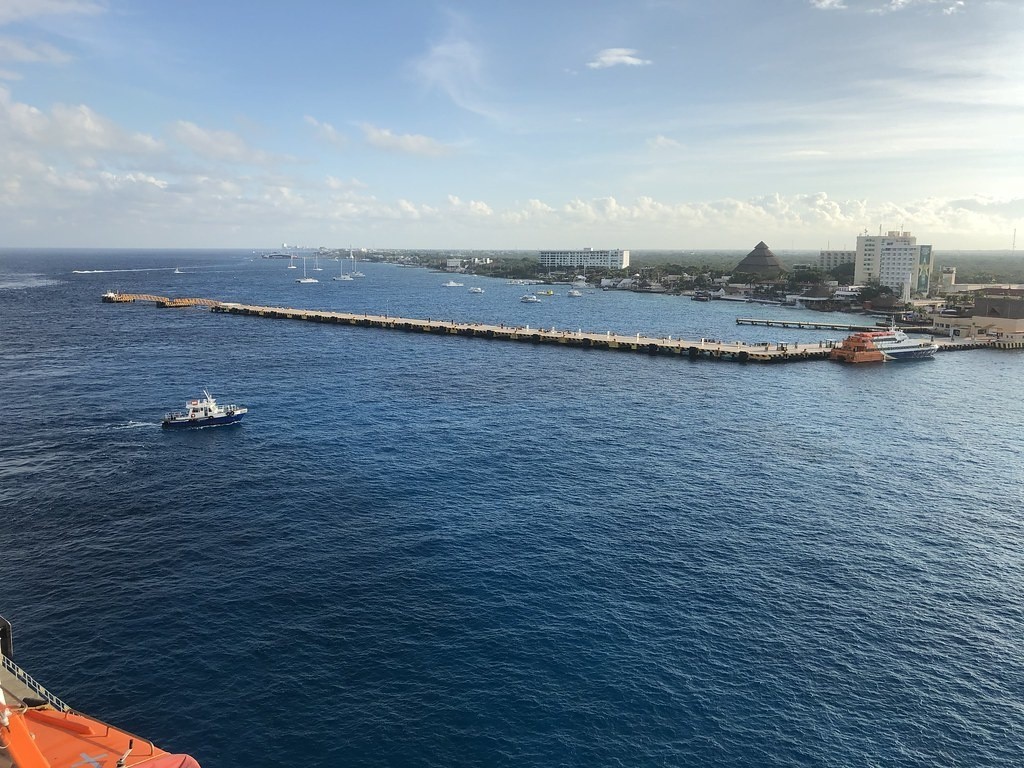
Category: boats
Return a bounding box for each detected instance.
[162,389,248,428]
[537,290,553,296]
[468,287,483,293]
[691,291,708,301]
[567,289,582,297]
[1,653,201,768]
[520,295,540,302]
[442,280,464,286]
[829,330,939,363]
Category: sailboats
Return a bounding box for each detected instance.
[174,268,180,274]
[288,253,366,284]
